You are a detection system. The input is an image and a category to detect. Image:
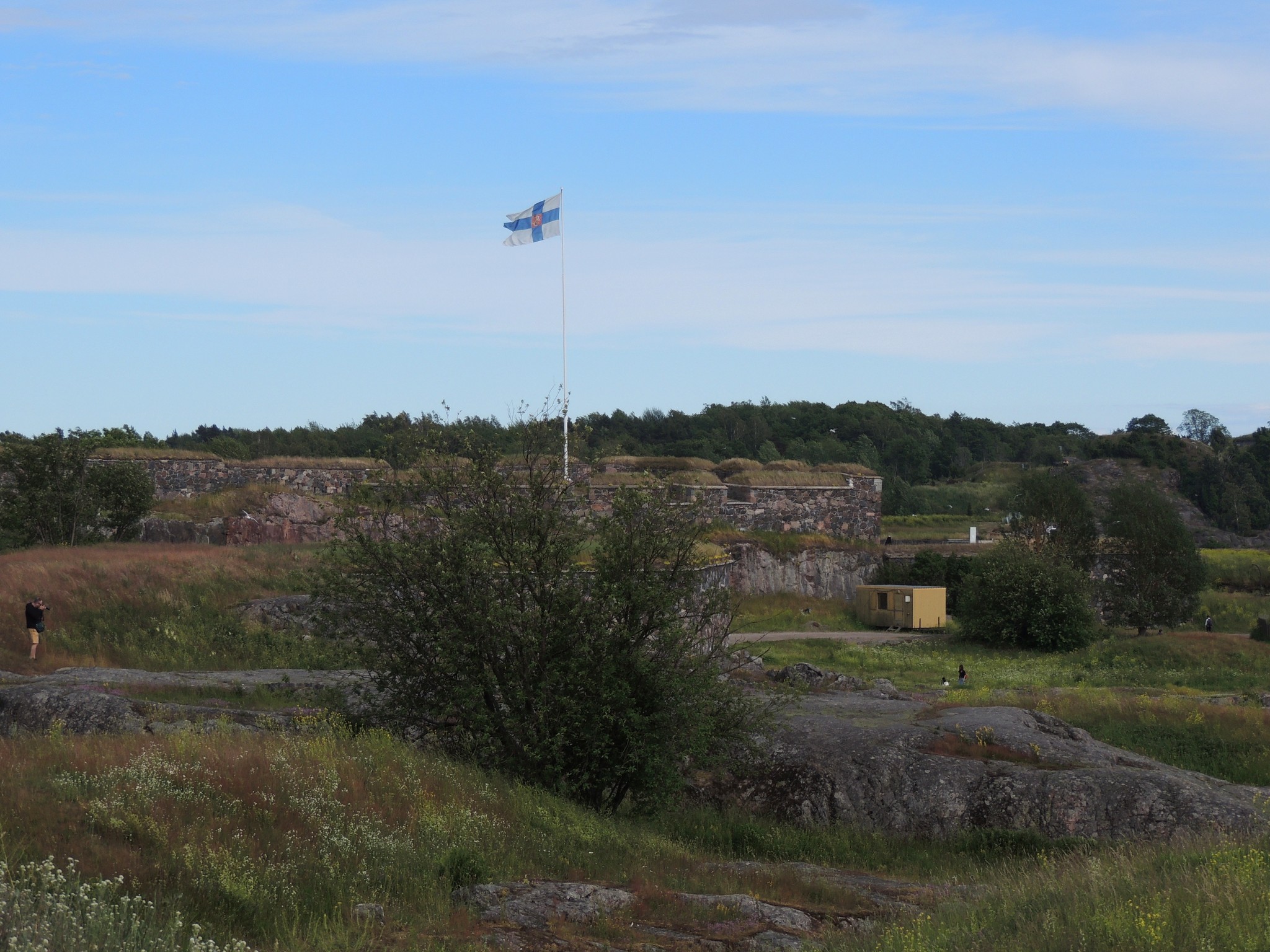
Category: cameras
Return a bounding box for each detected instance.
[42,605,50,610]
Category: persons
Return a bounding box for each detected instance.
[1063,460,1069,466]
[939,678,949,686]
[1204,615,1212,632]
[967,502,972,516]
[1157,629,1163,636]
[958,665,966,686]
[26,598,45,661]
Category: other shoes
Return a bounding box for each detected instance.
[28,657,37,664]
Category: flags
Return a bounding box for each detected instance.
[502,192,560,247]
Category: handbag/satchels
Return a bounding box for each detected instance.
[965,675,967,679]
[36,621,45,633]
